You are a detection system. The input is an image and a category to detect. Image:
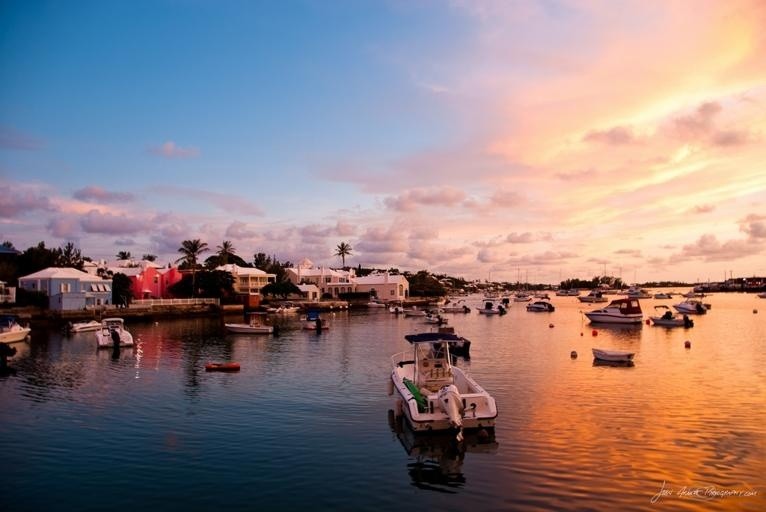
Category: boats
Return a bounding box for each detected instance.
[386,404,500,481]
[364,259,579,324]
[648,310,693,328]
[584,295,643,325]
[224,319,275,335]
[592,346,636,363]
[301,312,330,330]
[94,317,136,348]
[580,263,766,303]
[384,327,501,431]
[4,340,28,362]
[672,300,708,315]
[0,319,32,344]
[266,305,302,313]
[205,360,241,372]
[68,317,103,333]
